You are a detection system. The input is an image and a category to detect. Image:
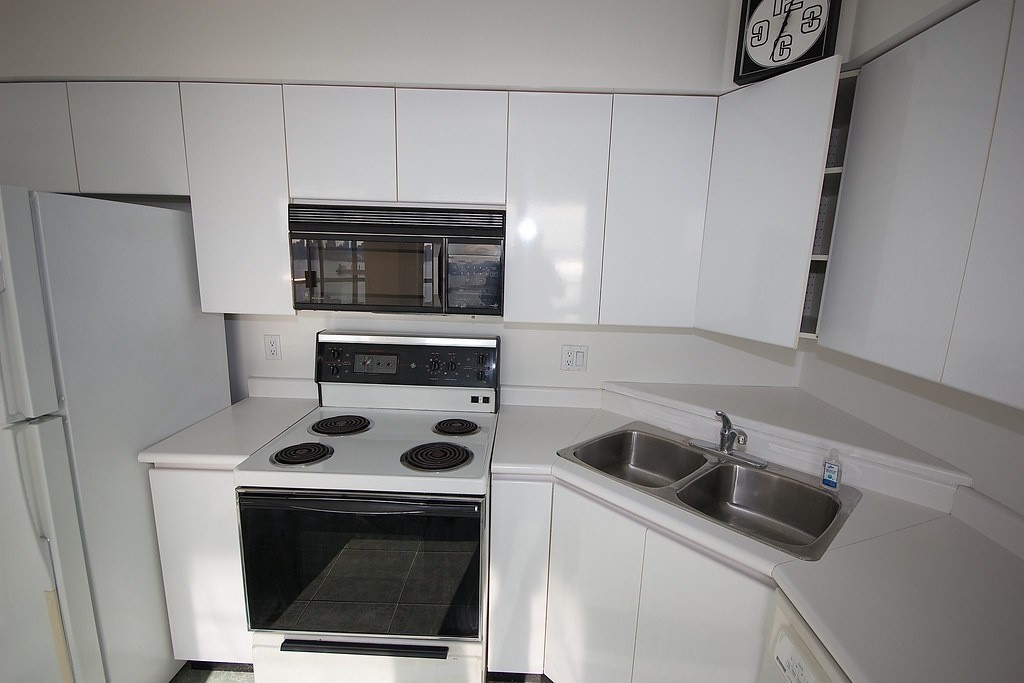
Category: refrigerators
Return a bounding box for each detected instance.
[0,183,233,680]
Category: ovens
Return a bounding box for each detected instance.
[236,490,485,645]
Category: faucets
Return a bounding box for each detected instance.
[715,409,747,454]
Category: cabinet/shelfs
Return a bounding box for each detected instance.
[484,474,552,674]
[180,81,297,317]
[692,52,861,353]
[147,462,253,663]
[502,91,719,328]
[810,1,1023,414]
[283,83,509,206]
[542,482,774,683]
[1,77,191,197]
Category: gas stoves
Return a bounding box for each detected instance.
[233,328,499,489]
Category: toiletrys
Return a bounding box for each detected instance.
[819,448,842,492]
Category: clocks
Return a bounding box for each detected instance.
[732,0,842,88]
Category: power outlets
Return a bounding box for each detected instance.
[561,344,589,372]
[264,334,281,360]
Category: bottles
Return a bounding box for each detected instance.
[820,447,843,493]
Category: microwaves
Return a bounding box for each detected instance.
[286,203,503,320]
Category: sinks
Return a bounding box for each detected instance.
[674,462,844,548]
[572,429,711,488]
